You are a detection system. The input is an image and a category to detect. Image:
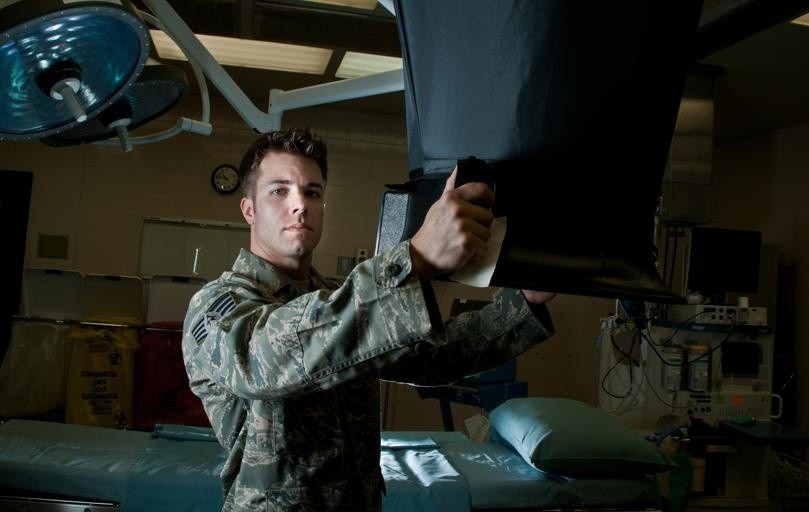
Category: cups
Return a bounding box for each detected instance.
[737,297,749,307]
[756,392,782,421]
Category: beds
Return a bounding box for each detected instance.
[1,418,675,510]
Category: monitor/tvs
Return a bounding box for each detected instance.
[685,226,760,304]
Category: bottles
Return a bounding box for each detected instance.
[662,345,685,391]
[691,458,706,493]
[687,344,709,392]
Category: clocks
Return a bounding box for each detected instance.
[209,160,242,196]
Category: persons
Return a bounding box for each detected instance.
[182,126,558,512]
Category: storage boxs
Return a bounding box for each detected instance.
[5,265,210,426]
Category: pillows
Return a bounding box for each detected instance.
[483,394,627,475]
